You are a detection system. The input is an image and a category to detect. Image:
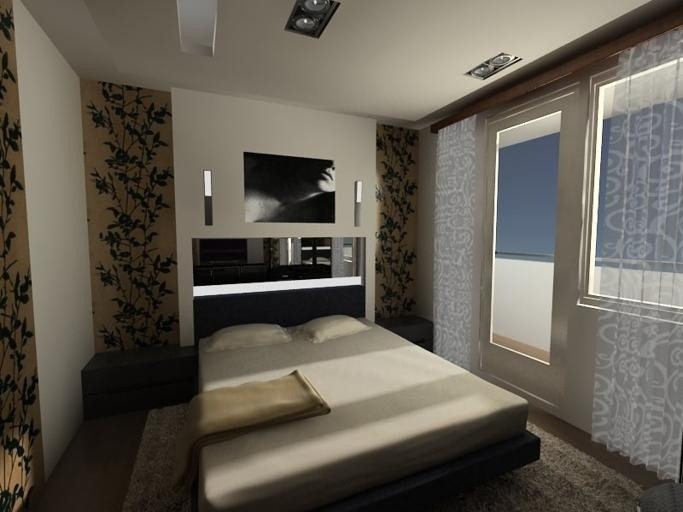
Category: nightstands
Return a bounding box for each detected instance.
[380,316,433,352]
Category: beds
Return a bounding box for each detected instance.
[194,316,540,511]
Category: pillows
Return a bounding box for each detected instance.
[206,315,373,351]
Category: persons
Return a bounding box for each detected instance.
[277,161,336,222]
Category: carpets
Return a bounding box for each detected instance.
[121,403,644,512]
